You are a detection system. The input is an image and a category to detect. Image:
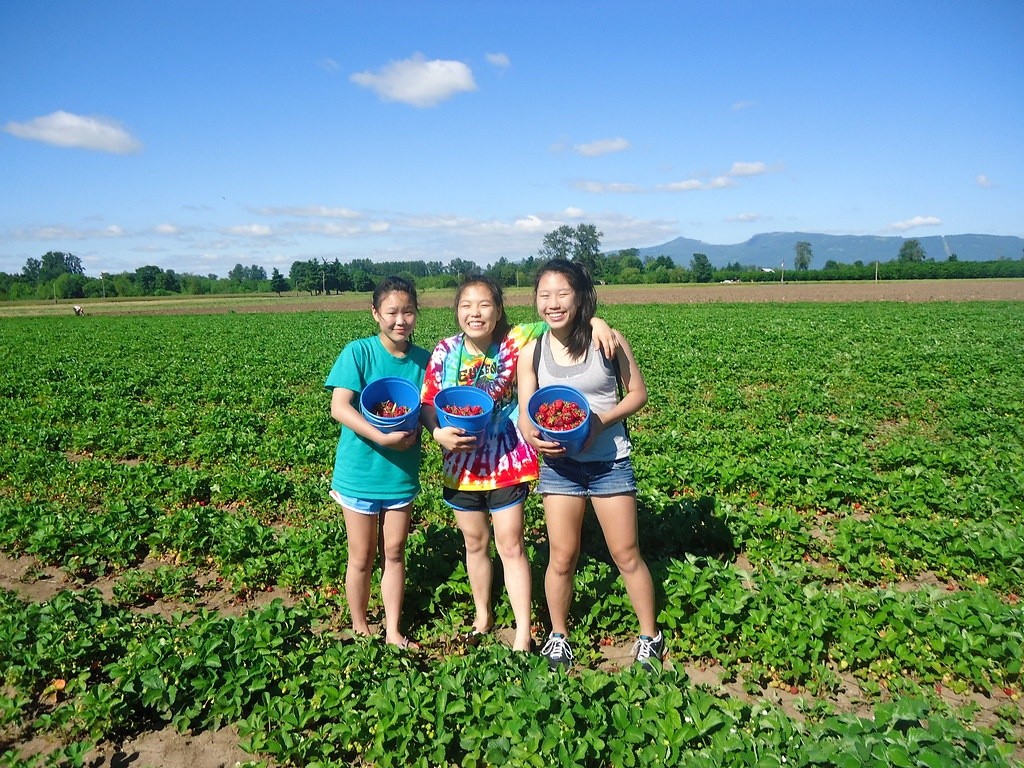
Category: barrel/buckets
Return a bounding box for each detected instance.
[433,385,494,452]
[527,384,592,457]
[357,376,422,445]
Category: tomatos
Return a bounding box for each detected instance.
[442,404,482,417]
[371,399,410,418]
[535,399,587,431]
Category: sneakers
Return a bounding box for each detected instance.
[633,630,664,678]
[543,629,574,675]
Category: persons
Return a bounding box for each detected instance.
[420,275,619,653]
[516,258,664,670]
[72,305,83,315]
[323,276,432,652]
